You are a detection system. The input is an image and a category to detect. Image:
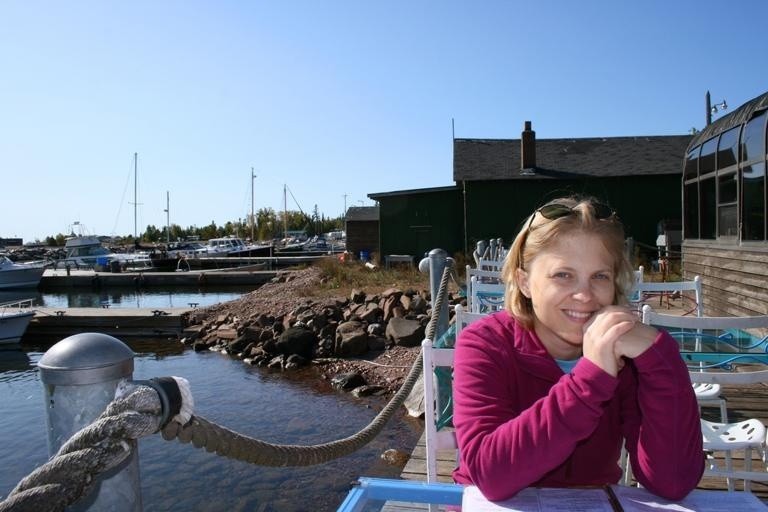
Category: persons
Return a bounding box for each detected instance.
[444,192,704,503]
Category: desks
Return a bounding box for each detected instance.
[337,477,768,512]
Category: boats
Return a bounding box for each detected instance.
[0,252,46,289]
[0,296,37,344]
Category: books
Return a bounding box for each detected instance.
[457,483,616,512]
[608,482,767,512]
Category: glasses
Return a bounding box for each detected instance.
[526,202,615,231]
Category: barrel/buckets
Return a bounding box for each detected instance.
[360,251,368,260]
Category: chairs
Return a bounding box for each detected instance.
[421,250,768,512]
